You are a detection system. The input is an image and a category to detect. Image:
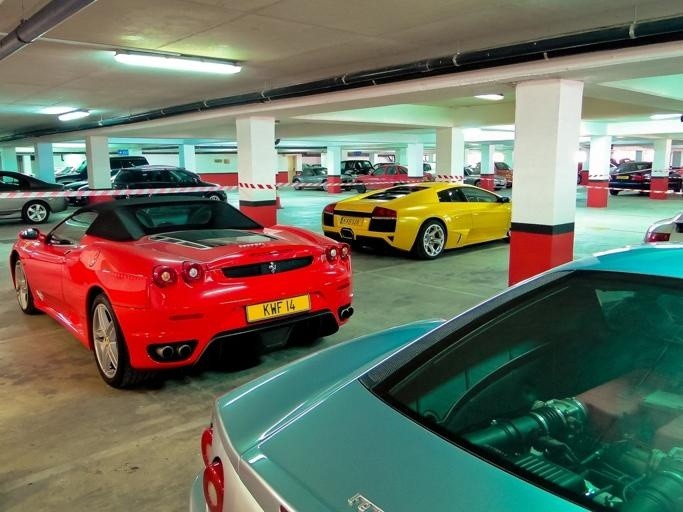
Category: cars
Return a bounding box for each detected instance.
[291,160,434,193]
[0,170,71,226]
[607,161,683,196]
[54,153,228,209]
[464,161,513,191]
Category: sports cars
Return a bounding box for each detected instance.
[321,180,512,261]
[8,193,354,391]
